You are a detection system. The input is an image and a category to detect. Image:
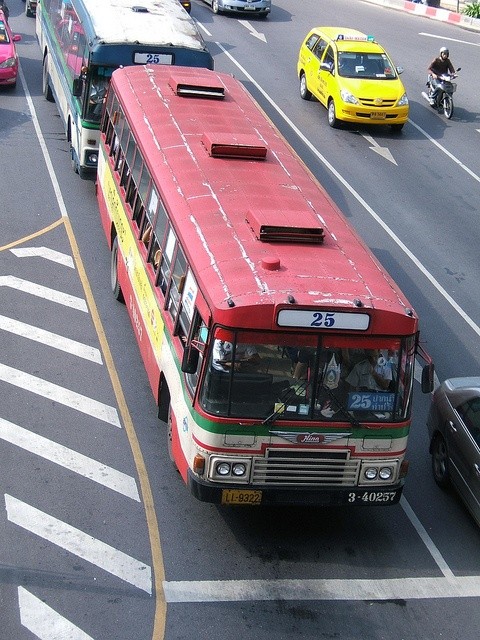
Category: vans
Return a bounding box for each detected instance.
[297,26,410,128]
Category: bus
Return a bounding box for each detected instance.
[94,62,435,511]
[35,1,214,176]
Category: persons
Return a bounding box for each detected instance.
[427,47,457,107]
[212,339,262,379]
[141,222,152,241]
[346,349,392,393]
[89,73,109,104]
[294,347,341,387]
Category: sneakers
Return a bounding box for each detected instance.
[430,97,435,105]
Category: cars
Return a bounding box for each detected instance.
[204,0,272,17]
[0,10,22,87]
[425,377,479,529]
[23,0,38,17]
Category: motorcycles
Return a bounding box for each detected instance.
[421,68,462,120]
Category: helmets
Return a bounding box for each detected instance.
[440,47,449,53]
